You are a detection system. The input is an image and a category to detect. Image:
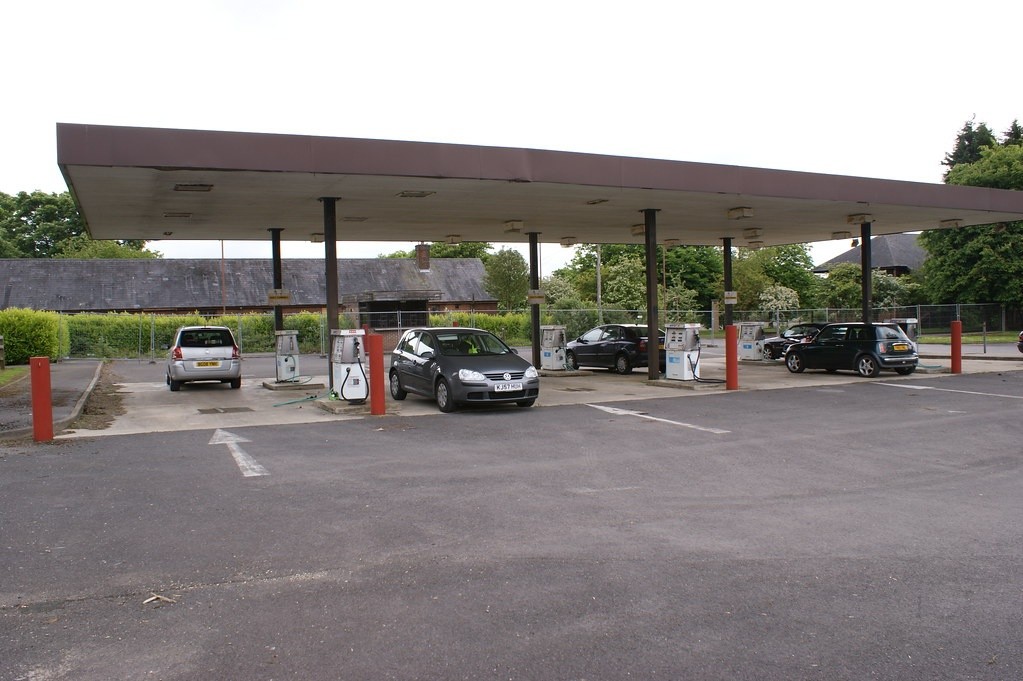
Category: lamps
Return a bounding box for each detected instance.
[940,219,963,229]
[830,231,851,241]
[504,220,523,233]
[848,212,872,226]
[729,208,754,220]
[560,237,577,247]
[445,234,463,244]
[746,242,765,251]
[744,227,764,240]
[311,234,325,243]
[630,223,645,237]
[664,238,680,248]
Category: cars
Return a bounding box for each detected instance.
[388,327,541,413]
[566,323,666,376]
[165,324,242,393]
[762,322,831,360]
[785,323,918,378]
[1016,330,1023,354]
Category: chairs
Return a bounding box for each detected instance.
[210,336,220,340]
[850,329,867,340]
[460,340,475,352]
[184,335,196,345]
